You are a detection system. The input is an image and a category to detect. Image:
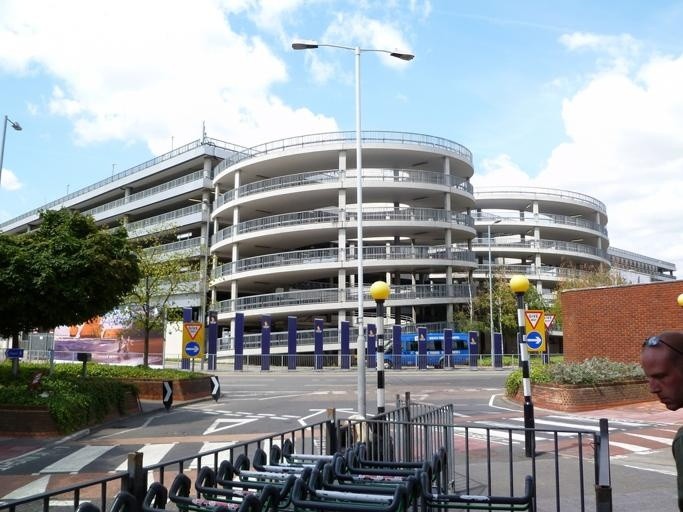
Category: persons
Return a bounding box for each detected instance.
[642,333,683,512]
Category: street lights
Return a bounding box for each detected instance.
[292,42,414,418]
[0,116,22,181]
[369,280,390,415]
[510,275,536,458]
[202,199,210,326]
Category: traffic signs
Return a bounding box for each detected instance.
[5,349,24,359]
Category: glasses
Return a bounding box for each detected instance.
[641,334,683,357]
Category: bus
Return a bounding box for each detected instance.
[384,332,469,369]
